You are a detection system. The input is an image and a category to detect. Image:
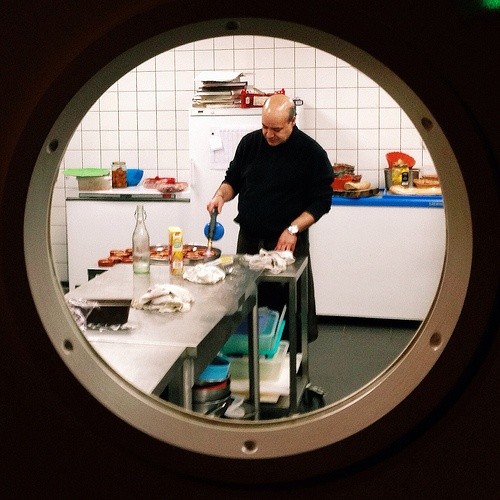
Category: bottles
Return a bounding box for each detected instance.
[111,162,127,187]
[132,204,151,274]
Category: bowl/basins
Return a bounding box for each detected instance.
[192,353,244,420]
[332,174,353,190]
[143,177,188,192]
[126,169,144,186]
[385,152,416,169]
[413,176,440,188]
[77,177,113,191]
[80,298,133,326]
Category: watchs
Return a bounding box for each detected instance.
[288,225,300,236]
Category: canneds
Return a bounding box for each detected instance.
[111,161,126,188]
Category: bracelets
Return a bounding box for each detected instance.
[217,195,224,198]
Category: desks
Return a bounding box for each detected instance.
[87,255,326,418]
[64,263,266,422]
[88,342,189,408]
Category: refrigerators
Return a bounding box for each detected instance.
[188,106,304,255]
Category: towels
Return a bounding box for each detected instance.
[139,282,194,313]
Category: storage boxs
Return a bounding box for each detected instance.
[76,174,112,190]
[217,310,290,380]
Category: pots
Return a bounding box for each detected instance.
[384,168,420,190]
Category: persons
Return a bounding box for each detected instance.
[207,93,335,378]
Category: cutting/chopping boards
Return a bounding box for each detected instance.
[333,188,380,198]
